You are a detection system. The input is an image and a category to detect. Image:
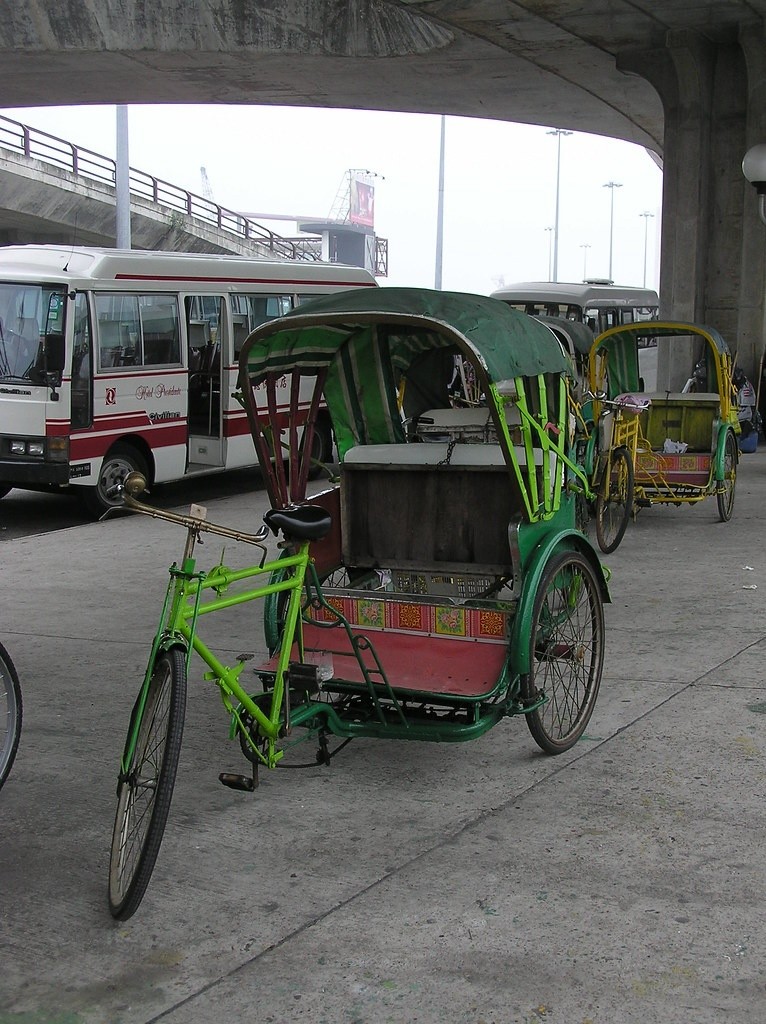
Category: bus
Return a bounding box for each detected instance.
[488,277,661,374]
[1,244,382,519]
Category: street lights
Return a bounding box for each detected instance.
[545,128,574,283]
[637,210,655,288]
[579,243,592,283]
[602,181,624,280]
[543,226,555,282]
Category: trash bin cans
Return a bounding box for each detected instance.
[617,391,724,452]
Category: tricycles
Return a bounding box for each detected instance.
[530,314,741,555]
[106,283,611,921]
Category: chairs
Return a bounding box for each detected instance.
[613,391,719,451]
[418,407,524,447]
[98,313,250,363]
[12,317,40,354]
[334,440,566,577]
